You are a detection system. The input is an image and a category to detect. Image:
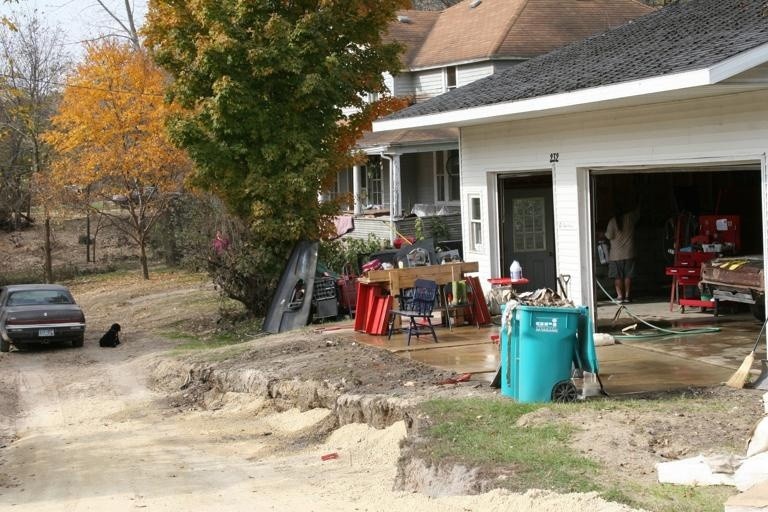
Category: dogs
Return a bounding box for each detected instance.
[100,323,121,347]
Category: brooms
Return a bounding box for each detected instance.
[726,317,768,390]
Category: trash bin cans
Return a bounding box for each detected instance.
[500,303,601,407]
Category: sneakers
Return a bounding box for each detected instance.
[612,297,624,302]
[624,297,632,303]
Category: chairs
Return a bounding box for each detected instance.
[387,279,439,346]
[442,281,477,332]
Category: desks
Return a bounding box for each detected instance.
[486,277,530,307]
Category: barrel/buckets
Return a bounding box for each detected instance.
[510,258,523,280]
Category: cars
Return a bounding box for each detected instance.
[0,284,87,350]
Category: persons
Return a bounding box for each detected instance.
[606,194,644,305]
[209,229,228,289]
